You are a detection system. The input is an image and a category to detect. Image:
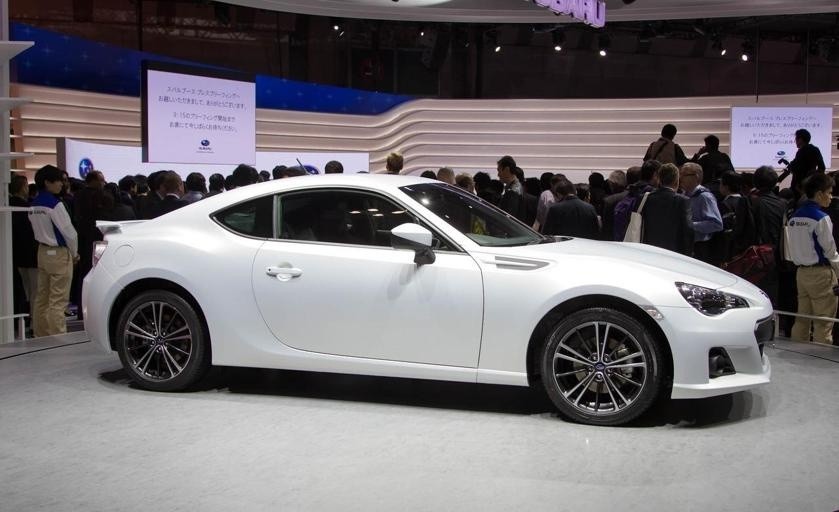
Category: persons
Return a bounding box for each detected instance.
[6,163,138,337]
[714,165,838,345]
[421,155,723,260]
[642,124,689,167]
[133,161,344,220]
[385,153,403,175]
[777,127,827,193]
[691,134,734,173]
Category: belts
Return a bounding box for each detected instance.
[800,263,830,267]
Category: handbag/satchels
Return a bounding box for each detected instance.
[621,189,649,243]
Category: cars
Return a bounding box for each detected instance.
[80,174,778,425]
